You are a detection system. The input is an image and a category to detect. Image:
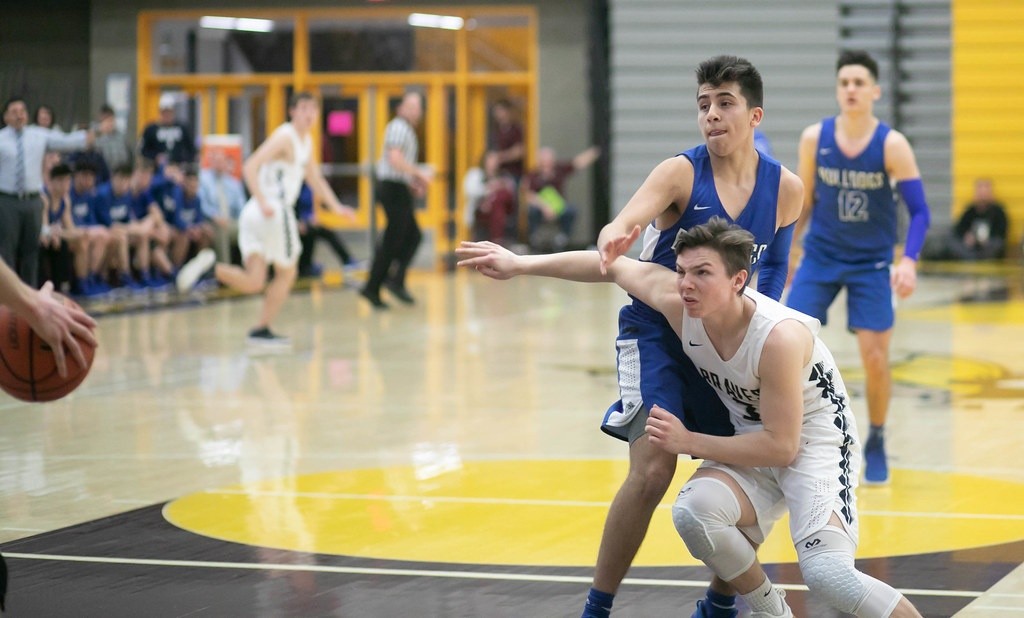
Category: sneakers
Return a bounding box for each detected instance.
[176,248,217,294]
[864,444,888,485]
[73,276,172,300]
[246,327,290,344]
[691,599,738,618]
[750,588,796,618]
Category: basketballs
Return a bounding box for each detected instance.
[0,290,98,404]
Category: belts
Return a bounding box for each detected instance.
[0,192,39,200]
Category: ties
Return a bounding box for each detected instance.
[16,131,25,202]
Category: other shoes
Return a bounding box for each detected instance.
[387,284,415,303]
[359,288,387,308]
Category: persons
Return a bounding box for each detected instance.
[797,47,929,483]
[582,56,806,616]
[934,176,1008,259]
[174,88,346,340]
[454,216,925,618]
[461,98,601,255]
[1,258,98,380]
[361,90,434,310]
[0,92,366,299]
